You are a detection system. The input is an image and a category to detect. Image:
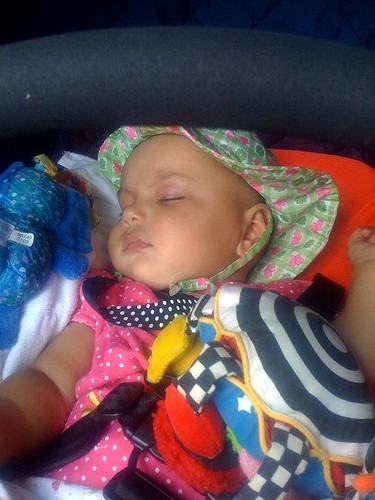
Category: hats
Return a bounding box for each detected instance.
[96,127,339,295]
